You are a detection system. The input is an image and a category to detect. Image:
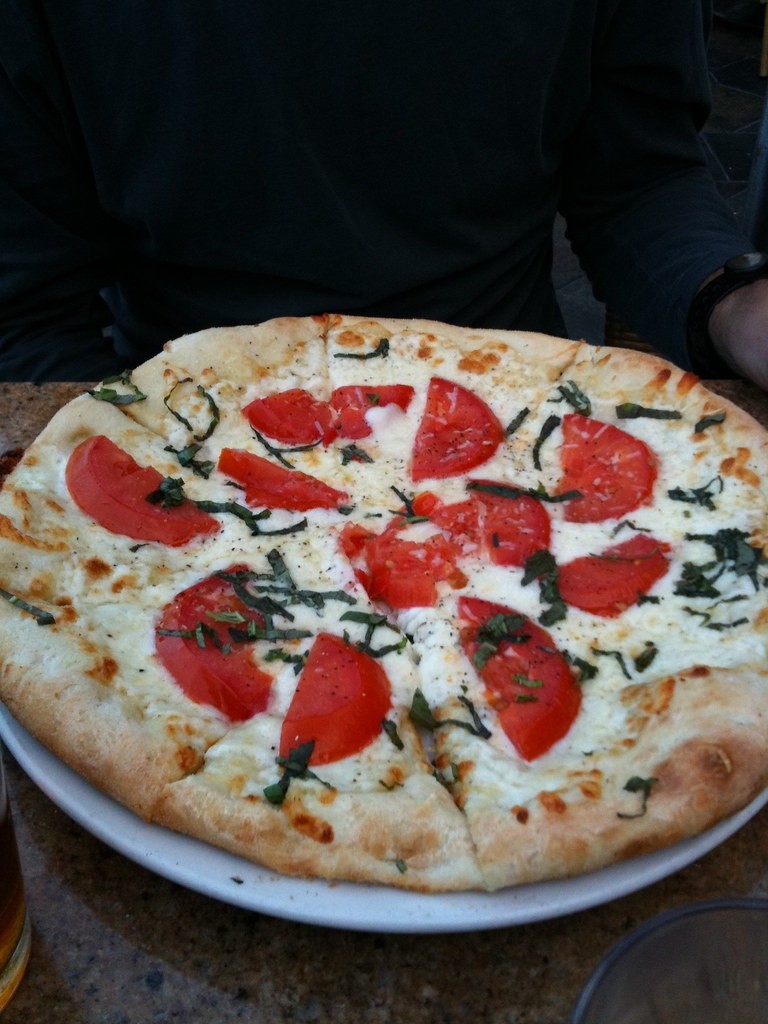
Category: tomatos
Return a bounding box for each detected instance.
[66,376,666,772]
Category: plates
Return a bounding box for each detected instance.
[0,701,768,934]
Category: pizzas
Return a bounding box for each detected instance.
[0,313,767,893]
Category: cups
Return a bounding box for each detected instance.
[0,754,30,1013]
[573,897,768,1024]
